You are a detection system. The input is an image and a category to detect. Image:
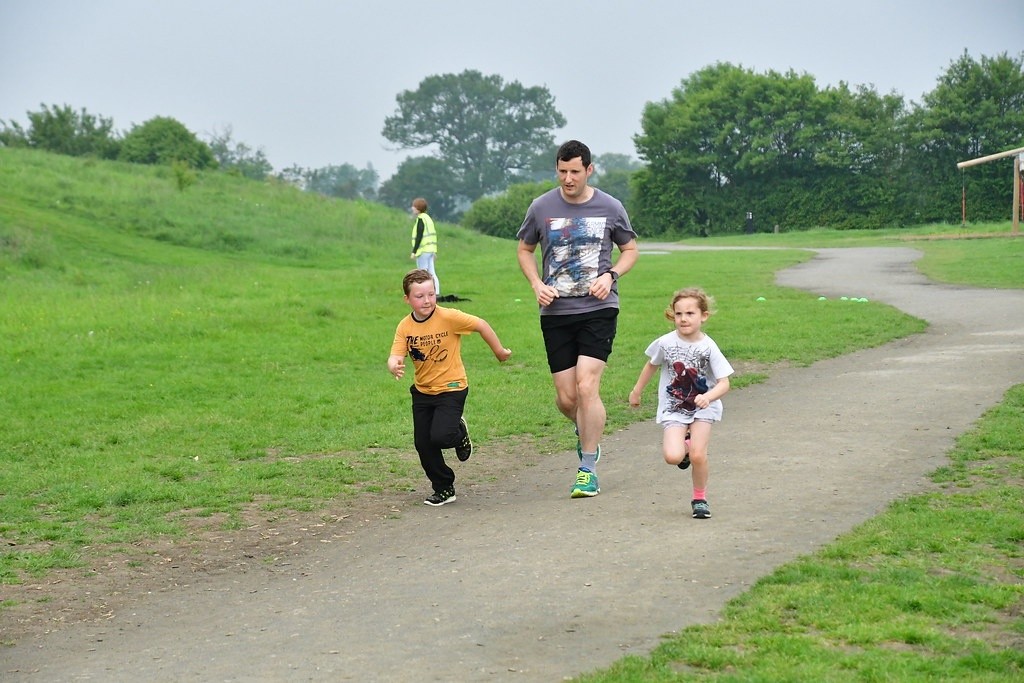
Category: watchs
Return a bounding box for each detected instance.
[604,270,619,283]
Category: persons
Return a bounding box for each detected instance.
[629,286,735,519]
[410,197,439,296]
[515,140,638,498]
[387,268,512,506]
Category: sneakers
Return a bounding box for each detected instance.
[455,418,472,462]
[691,498,712,518]
[575,425,600,463]
[678,430,692,469]
[424,485,456,506]
[571,466,600,499]
